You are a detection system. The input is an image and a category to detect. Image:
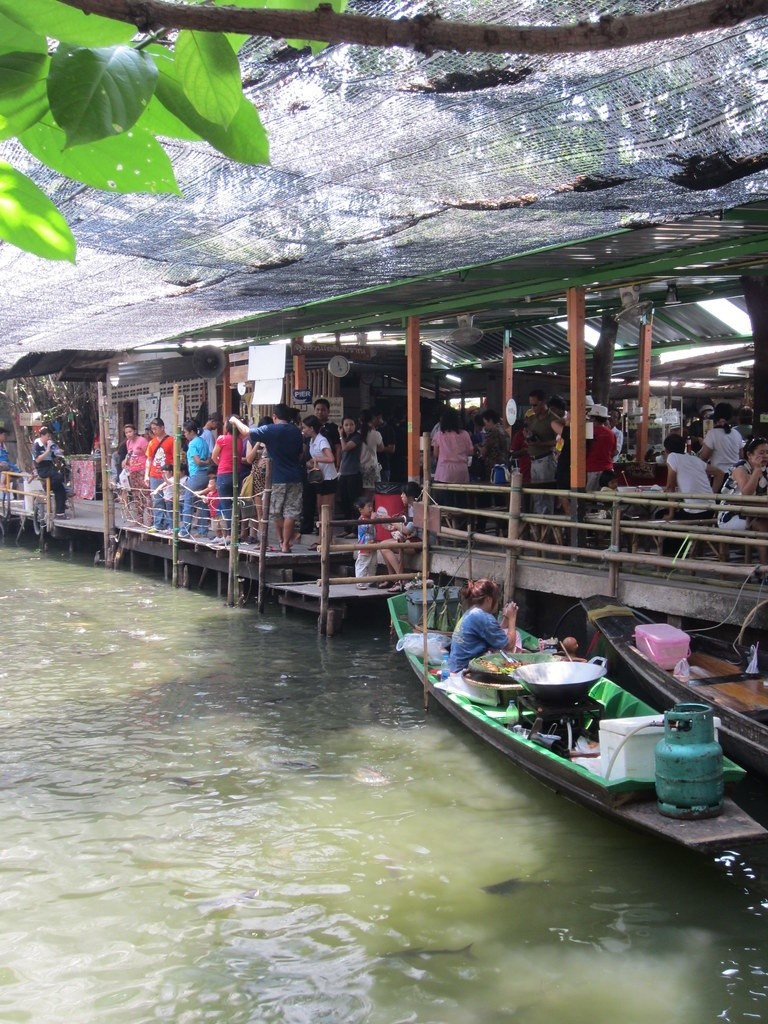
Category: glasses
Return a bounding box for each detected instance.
[747,436,766,447]
[530,403,538,407]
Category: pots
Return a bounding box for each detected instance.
[508,657,607,704]
[468,652,556,684]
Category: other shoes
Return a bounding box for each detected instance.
[55,514,71,520]
[148,526,208,540]
[211,534,258,545]
[336,531,358,539]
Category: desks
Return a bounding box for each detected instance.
[614,485,680,554]
[73,460,102,501]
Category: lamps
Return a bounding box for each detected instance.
[664,283,682,305]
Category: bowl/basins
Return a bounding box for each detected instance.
[639,485,663,491]
[617,486,636,493]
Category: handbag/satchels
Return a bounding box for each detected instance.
[489,464,510,486]
[240,475,254,503]
[306,468,325,486]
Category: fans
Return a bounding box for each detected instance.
[190,345,225,380]
[615,287,653,324]
[449,315,483,345]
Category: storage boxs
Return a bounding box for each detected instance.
[408,585,462,625]
[597,714,721,782]
[634,623,690,669]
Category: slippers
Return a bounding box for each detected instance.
[379,580,409,592]
[307,542,321,551]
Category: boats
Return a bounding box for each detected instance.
[579,593,768,784]
[387,589,768,852]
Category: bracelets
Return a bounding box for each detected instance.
[503,614,508,619]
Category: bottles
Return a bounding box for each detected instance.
[492,463,506,484]
[440,655,450,682]
[506,700,520,732]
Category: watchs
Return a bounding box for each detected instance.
[339,437,344,440]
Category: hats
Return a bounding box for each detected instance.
[588,405,611,418]
[525,409,536,418]
[585,394,595,408]
[149,418,165,426]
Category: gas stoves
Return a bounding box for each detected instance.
[515,693,605,735]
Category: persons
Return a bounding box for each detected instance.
[354,496,378,590]
[212,413,273,547]
[144,417,189,532]
[290,398,396,551]
[657,434,725,566]
[110,423,155,528]
[173,413,224,538]
[378,481,437,592]
[0,427,22,494]
[430,406,510,548]
[688,403,754,502]
[511,387,624,562]
[717,436,768,586]
[450,579,519,673]
[229,404,305,552]
[33,427,71,519]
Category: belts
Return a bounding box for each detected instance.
[530,451,553,461]
